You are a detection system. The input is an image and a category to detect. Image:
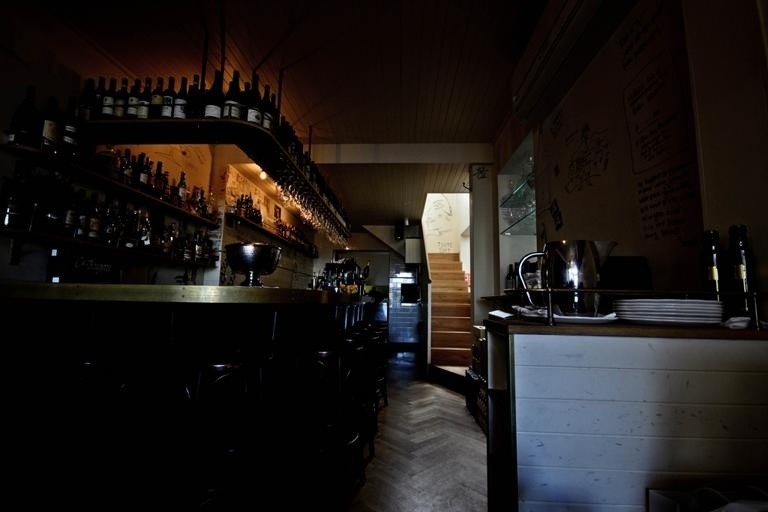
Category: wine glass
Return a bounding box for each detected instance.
[226,243,281,287]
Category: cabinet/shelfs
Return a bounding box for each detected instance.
[497,130,535,295]
[485,330,518,512]
[0,115,353,285]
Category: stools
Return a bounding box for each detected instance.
[184,318,391,489]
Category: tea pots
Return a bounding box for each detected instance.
[517,239,618,316]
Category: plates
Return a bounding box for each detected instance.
[612,299,723,325]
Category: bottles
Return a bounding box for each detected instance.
[505,262,519,289]
[2,70,343,260]
[703,225,752,318]
